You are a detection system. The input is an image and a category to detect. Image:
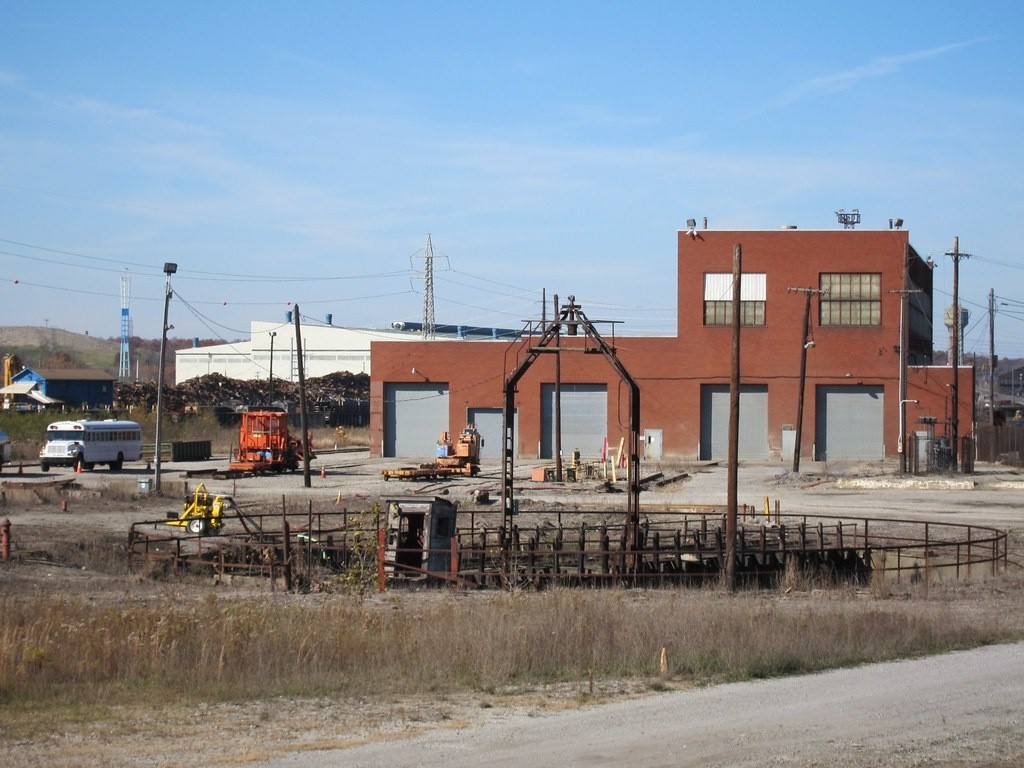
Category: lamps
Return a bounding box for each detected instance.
[895,218,903,229]
[411,368,417,374]
[687,219,696,229]
[846,371,852,377]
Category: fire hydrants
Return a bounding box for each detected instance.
[0,517,12,560]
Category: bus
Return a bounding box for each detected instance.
[39,418,143,470]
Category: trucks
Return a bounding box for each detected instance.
[382,422,484,480]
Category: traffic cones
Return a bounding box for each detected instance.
[321,465,326,478]
[18,462,23,476]
[146,462,151,470]
[76,461,82,474]
[337,491,341,504]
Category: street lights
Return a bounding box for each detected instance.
[269,332,276,409]
[153,262,179,490]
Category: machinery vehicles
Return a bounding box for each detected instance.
[231,411,318,473]
[163,483,267,542]
